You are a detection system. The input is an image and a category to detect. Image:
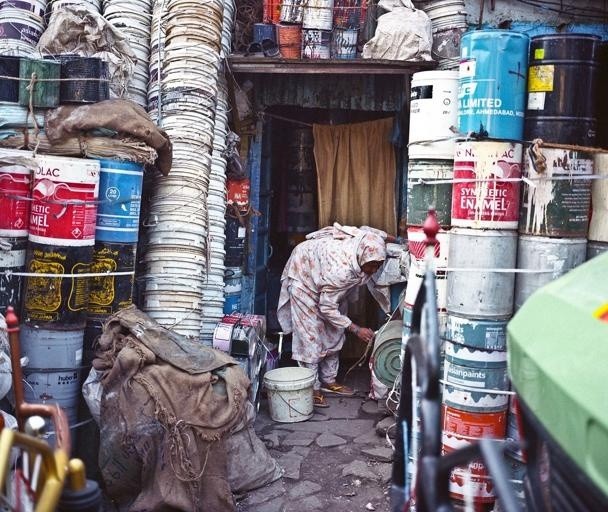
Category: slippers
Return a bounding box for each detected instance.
[313,394,330,408]
[321,384,356,395]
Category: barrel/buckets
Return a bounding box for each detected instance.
[0,0,608,512]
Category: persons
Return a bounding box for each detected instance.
[274,226,406,408]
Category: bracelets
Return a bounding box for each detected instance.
[353,325,360,336]
